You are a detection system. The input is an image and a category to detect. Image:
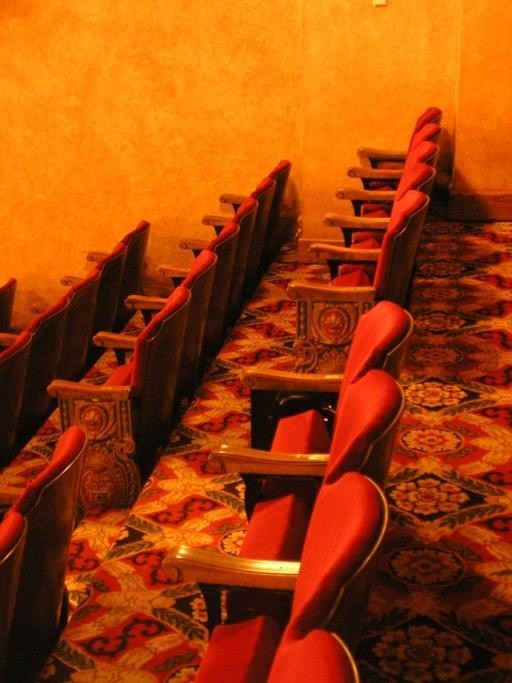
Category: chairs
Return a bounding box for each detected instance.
[178,473,388,679]
[346,105,444,190]
[182,367,407,632]
[0,158,293,679]
[213,299,417,472]
[286,191,430,366]
[271,626,360,683]
[334,123,441,213]
[310,164,438,278]
[322,141,440,246]
